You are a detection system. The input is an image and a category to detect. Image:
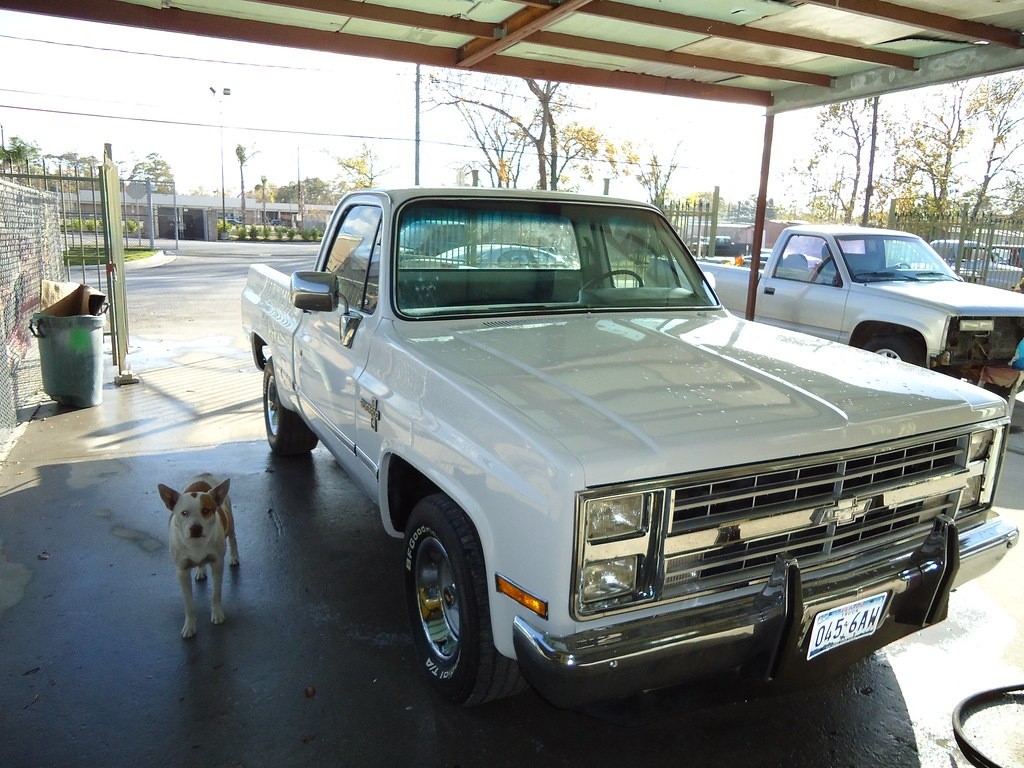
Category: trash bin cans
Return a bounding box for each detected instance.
[28,312,108,409]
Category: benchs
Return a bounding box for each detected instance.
[376,277,582,313]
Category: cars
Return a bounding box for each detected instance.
[686,233,758,260]
[926,236,1023,294]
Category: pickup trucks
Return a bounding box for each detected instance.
[234,182,1021,689]
[641,222,1022,386]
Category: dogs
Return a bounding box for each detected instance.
[156,472,240,639]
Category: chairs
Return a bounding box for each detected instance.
[780,254,813,284]
[852,253,882,282]
